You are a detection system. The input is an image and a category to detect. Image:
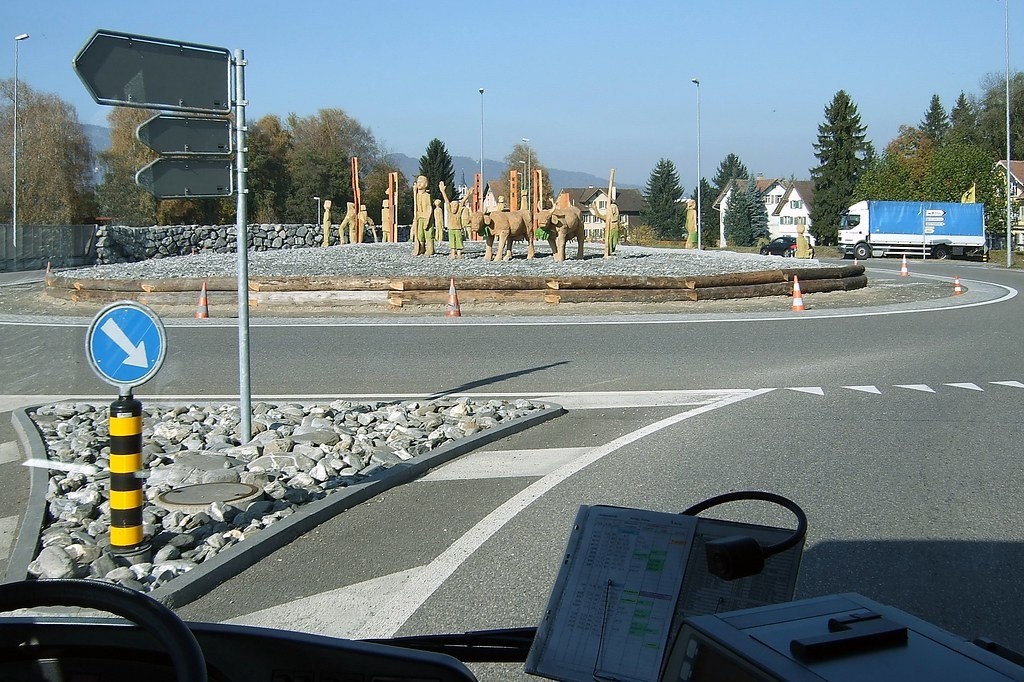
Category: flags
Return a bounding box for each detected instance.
[961,186,975,204]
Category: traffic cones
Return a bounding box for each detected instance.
[790,274,805,311]
[899,252,908,276]
[195,281,209,320]
[445,277,461,317]
[953,274,962,294]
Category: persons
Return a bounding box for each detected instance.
[757,233,769,246]
[412,175,556,259]
[590,203,621,253]
[795,224,813,258]
[685,199,697,249]
[320,199,393,247]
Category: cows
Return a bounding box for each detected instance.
[534,207,585,262]
[465,210,533,263]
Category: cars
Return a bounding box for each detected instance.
[759,237,814,259]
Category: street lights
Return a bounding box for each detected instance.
[478,88,484,213]
[519,162,526,192]
[314,197,320,225]
[12,34,29,276]
[523,137,530,212]
[691,79,702,250]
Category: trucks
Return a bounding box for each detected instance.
[836,199,986,260]
